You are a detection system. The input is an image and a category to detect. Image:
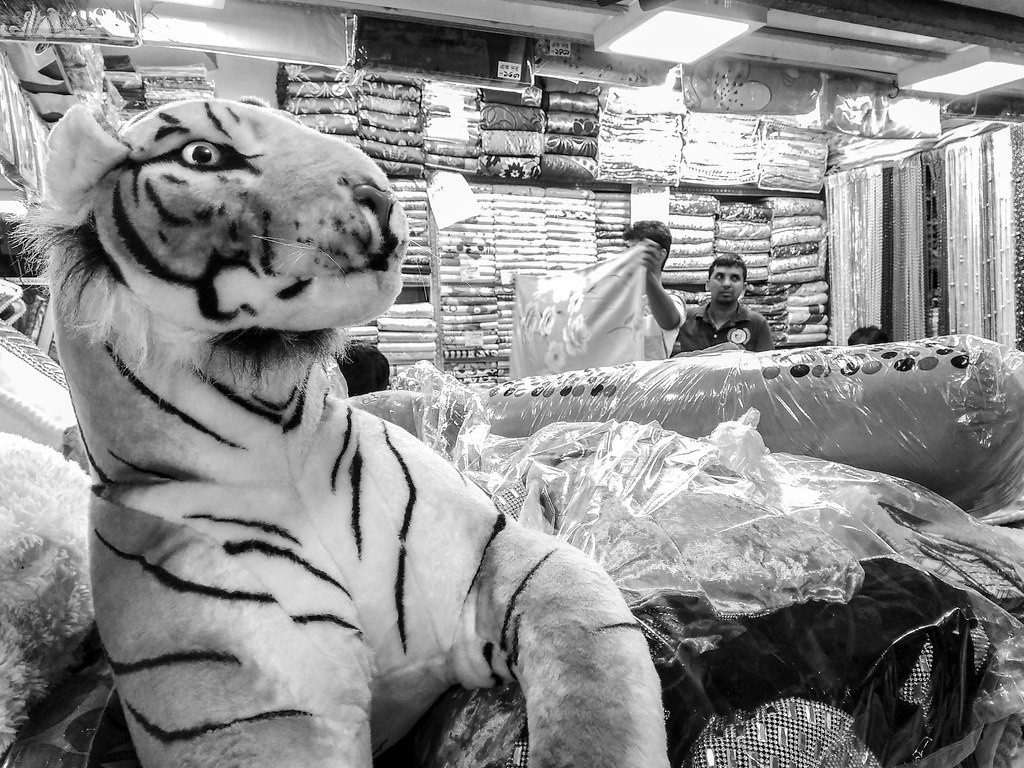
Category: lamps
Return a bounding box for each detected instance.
[594,0,767,64]
[897,46,1024,96]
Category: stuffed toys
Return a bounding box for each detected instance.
[10,93,672,768]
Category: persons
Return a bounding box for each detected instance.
[334,343,391,395]
[848,325,889,346]
[674,251,776,357]
[512,219,688,361]
